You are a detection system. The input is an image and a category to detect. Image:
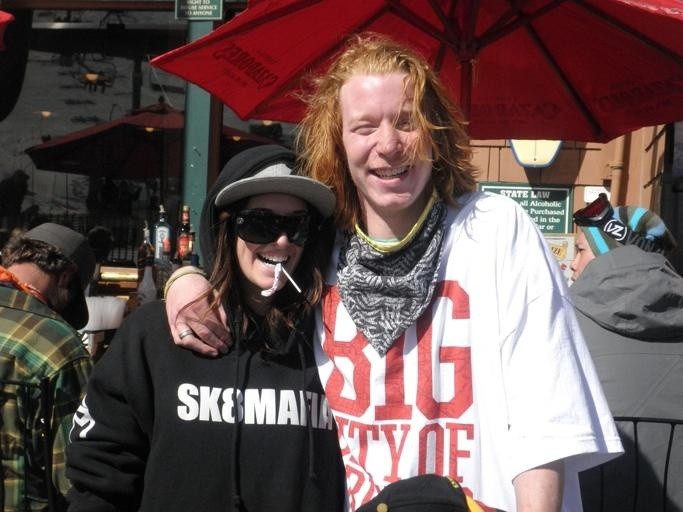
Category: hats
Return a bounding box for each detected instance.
[20,221,98,332]
[212,159,338,223]
[580,201,678,260]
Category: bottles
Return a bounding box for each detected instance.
[154,238,176,301]
[137,261,157,307]
[136,220,156,290]
[181,231,200,268]
[175,205,193,259]
[153,204,175,263]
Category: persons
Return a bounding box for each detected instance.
[162,36,627,510]
[60,146,347,510]
[556,205,681,512]
[0,222,99,512]
[83,225,120,296]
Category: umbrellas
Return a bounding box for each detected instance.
[22,94,287,177]
[147,1,681,147]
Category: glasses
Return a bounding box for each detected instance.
[571,196,661,252]
[232,202,325,247]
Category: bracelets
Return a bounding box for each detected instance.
[161,269,209,303]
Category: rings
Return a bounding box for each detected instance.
[178,329,193,339]
